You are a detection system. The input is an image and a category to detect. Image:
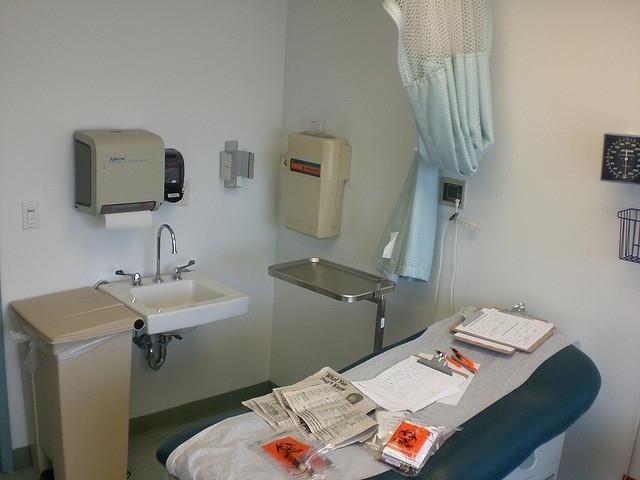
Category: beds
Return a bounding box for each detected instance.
[157,314,602,480]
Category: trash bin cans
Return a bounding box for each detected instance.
[9,286,146,480]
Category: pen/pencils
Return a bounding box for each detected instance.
[435,346,479,374]
[277,442,334,476]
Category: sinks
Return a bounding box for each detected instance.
[97,271,251,336]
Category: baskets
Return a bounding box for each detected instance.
[618,208,640,262]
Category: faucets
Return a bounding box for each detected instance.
[153,224,177,285]
